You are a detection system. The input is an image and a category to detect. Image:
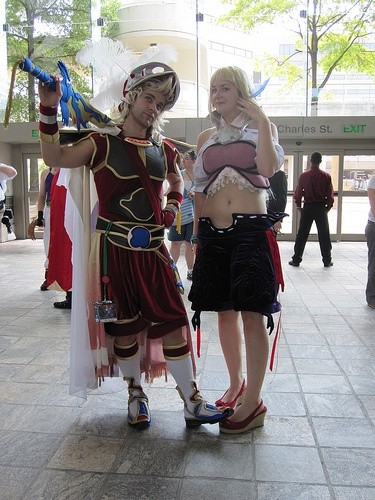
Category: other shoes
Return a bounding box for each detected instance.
[368,303,375,308]
[324,263,333,266]
[40,279,54,291]
[54,291,72,309]
[289,261,299,266]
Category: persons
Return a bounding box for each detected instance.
[0,163,17,241]
[187,63,290,435]
[36,166,60,291]
[38,63,234,431]
[46,168,73,309]
[364,174,375,309]
[168,149,198,281]
[288,150,334,268]
[265,170,288,313]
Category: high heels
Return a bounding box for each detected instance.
[218,400,267,433]
[215,378,247,410]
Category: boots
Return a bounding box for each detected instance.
[176,382,234,428]
[124,376,151,425]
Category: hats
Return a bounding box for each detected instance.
[122,63,180,112]
